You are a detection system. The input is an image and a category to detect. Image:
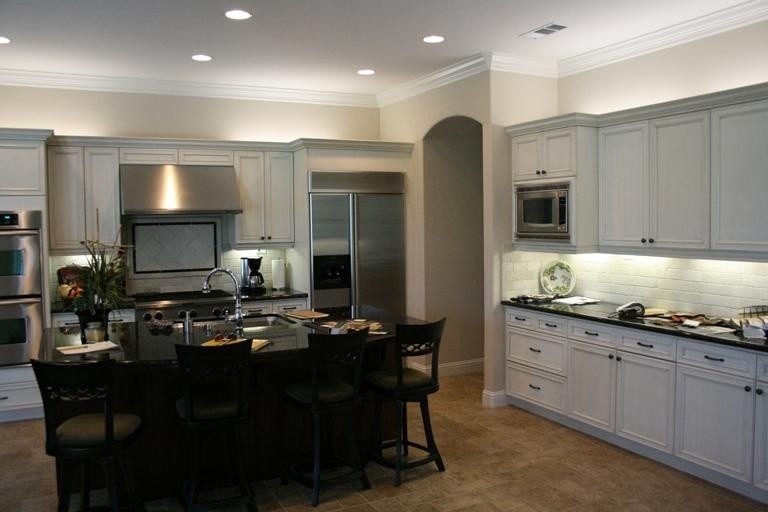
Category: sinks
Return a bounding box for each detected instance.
[173,312,298,328]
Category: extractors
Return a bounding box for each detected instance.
[119,163,243,218]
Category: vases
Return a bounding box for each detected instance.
[75,304,112,345]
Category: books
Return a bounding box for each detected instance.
[551,295,601,307]
[202,331,270,353]
[617,301,768,343]
[287,307,384,335]
[57,340,119,354]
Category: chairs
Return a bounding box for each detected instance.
[31,358,144,511]
[360,318,446,486]
[279,327,371,507]
[175,338,259,512]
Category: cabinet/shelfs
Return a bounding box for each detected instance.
[597,108,710,259]
[47,145,121,257]
[504,306,567,427]
[0,363,46,423]
[709,99,768,263]
[505,112,597,183]
[0,140,48,197]
[241,297,307,317]
[567,313,676,470]
[223,150,295,249]
[673,329,768,506]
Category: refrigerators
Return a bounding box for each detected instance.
[309,171,407,323]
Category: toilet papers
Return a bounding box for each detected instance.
[272,259,286,289]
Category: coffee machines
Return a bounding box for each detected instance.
[239,257,266,296]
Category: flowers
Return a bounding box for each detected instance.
[57,207,136,317]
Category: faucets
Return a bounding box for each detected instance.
[202,267,243,331]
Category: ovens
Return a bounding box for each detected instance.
[134,317,236,360]
[0,210,48,369]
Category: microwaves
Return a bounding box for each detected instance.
[514,189,569,239]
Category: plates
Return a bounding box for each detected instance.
[541,259,575,297]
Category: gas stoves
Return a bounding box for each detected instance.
[131,288,237,322]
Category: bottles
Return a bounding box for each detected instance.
[84,322,129,343]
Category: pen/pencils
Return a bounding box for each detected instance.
[56,346,87,351]
